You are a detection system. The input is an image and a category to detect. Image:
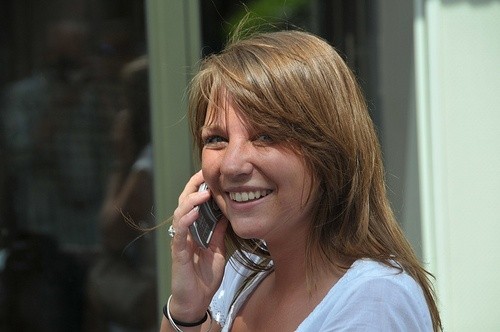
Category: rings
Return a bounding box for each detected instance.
[168,225,174,240]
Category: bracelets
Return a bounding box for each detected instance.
[162,294,214,332]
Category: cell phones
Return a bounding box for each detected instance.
[189,183,223,250]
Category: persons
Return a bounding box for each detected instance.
[160,30,444,332]
